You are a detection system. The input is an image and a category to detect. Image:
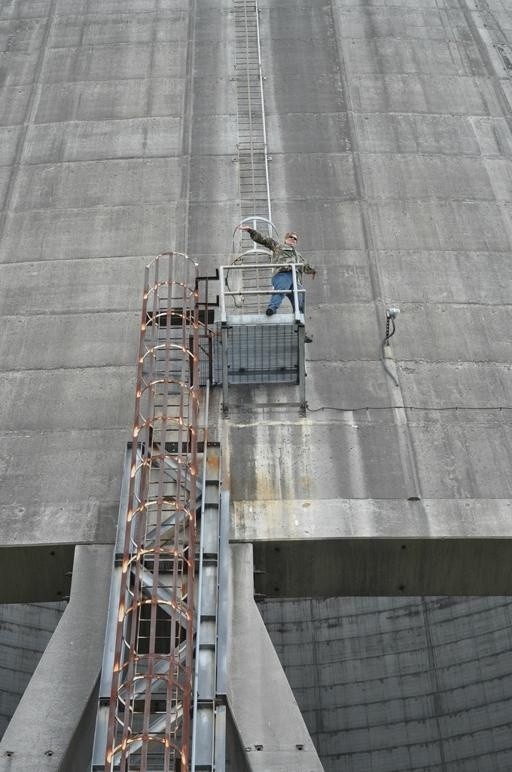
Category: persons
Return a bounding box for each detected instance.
[238,225,316,316]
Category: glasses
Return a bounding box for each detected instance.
[289,235,296,241]
